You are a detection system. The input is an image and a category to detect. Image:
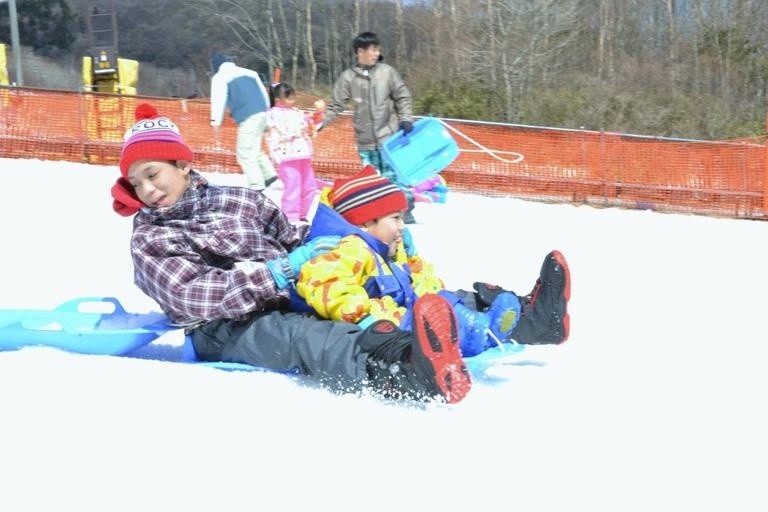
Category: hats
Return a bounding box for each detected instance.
[213,54,226,72]
[327,163,408,225]
[119,104,193,180]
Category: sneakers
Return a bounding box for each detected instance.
[434,289,523,357]
[249,175,278,192]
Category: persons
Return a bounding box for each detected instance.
[208,51,278,191]
[310,31,417,227]
[297,162,522,359]
[121,103,572,404]
[263,81,329,224]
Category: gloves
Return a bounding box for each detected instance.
[397,121,413,137]
[266,234,341,290]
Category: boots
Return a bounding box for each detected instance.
[471,250,571,345]
[357,293,472,405]
